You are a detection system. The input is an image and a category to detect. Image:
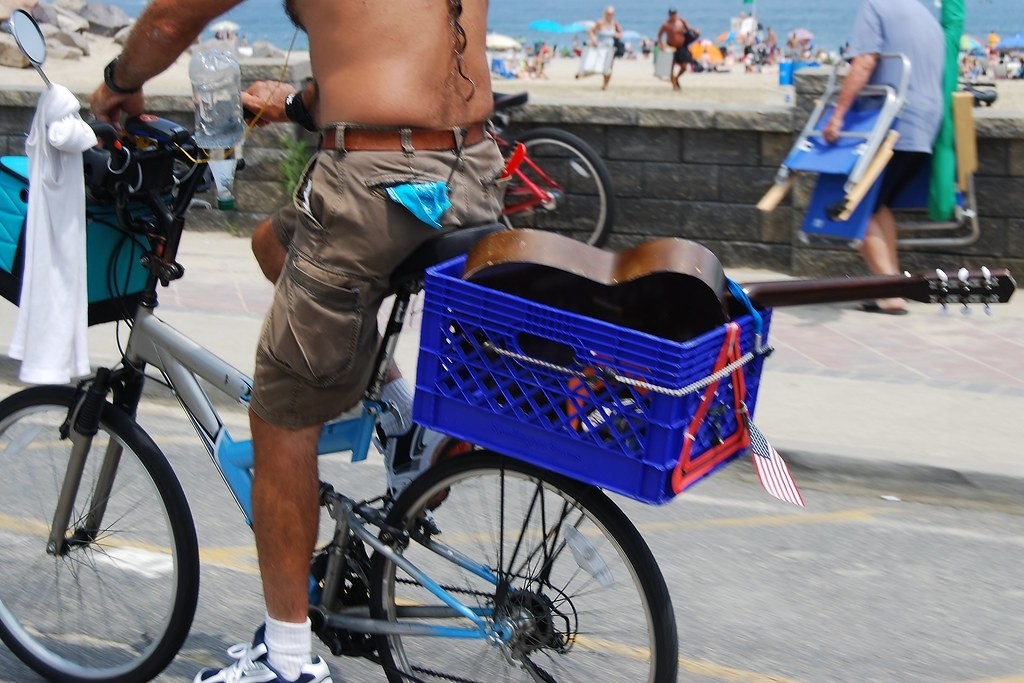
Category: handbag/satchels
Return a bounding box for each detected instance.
[681,19,698,43]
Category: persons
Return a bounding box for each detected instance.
[591,5,625,91]
[825,1,944,315]
[958,46,1024,82]
[701,12,825,72]
[658,7,701,92]
[89,0,508,683]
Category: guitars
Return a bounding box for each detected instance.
[458,223,1019,356]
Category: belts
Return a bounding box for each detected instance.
[318,121,486,151]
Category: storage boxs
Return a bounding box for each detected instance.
[408,253,776,508]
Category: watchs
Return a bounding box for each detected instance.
[104,51,144,94]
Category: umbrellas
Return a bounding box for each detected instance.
[527,18,641,41]
[485,32,522,53]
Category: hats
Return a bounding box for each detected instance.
[669,9,677,14]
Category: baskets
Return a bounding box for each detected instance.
[411,249,774,506]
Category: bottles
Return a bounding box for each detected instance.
[189,52,245,209]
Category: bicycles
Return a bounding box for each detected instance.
[0,9,775,683]
[490,92,616,250]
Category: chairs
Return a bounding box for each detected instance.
[888,90,983,251]
[654,44,677,82]
[574,44,616,80]
[755,49,913,254]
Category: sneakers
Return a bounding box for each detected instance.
[193,622,332,683]
[371,415,474,518]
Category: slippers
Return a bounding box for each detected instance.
[860,302,909,315]
